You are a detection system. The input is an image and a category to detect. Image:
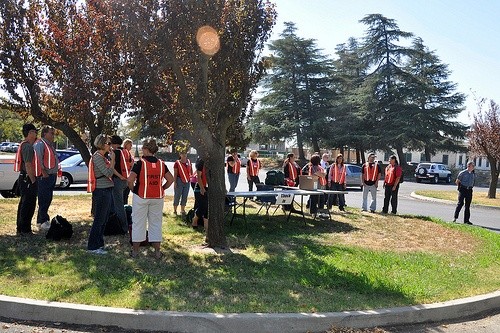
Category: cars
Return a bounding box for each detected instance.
[345,164,363,191]
[59,153,89,188]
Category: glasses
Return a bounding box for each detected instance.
[104,142,110,145]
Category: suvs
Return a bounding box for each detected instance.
[414,163,452,183]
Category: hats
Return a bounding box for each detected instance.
[23,124,40,131]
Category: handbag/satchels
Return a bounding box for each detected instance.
[104,214,121,236]
[46,215,74,241]
[266,170,279,185]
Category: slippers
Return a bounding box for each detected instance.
[130,251,138,258]
[156,250,163,259]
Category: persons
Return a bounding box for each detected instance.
[173,150,193,217]
[453,161,475,225]
[226,149,241,205]
[190,155,208,231]
[86,133,115,255]
[302,152,346,218]
[33,125,59,229]
[282,153,302,213]
[379,155,402,215]
[127,137,174,260]
[246,150,262,201]
[360,153,381,213]
[14,123,41,236]
[104,134,135,236]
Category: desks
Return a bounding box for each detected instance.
[226,192,324,230]
[273,186,348,222]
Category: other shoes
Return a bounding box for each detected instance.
[340,209,344,212]
[38,221,50,229]
[192,223,197,230]
[173,211,177,218]
[379,212,385,215]
[182,210,187,220]
[371,210,375,213]
[311,213,315,217]
[17,231,38,236]
[87,248,108,255]
[102,242,118,249]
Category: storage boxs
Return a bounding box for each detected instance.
[299,171,323,191]
